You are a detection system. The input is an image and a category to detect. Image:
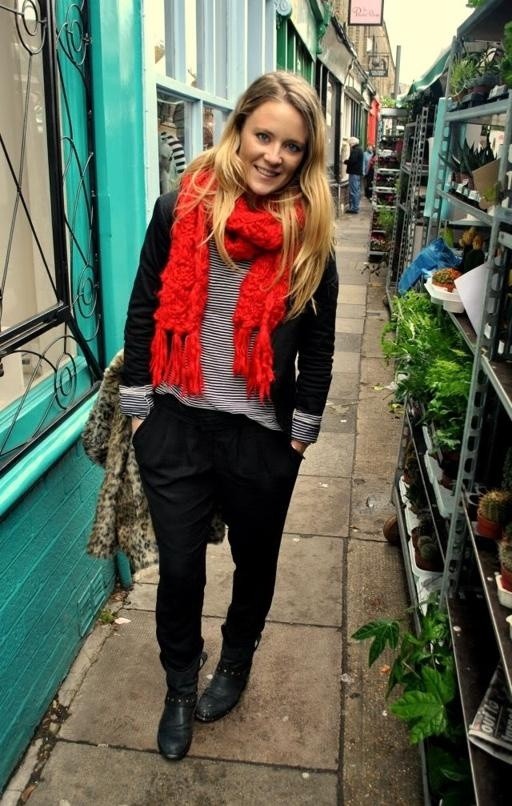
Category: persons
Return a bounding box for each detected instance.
[120,70,338,761]
[362,143,374,199]
[344,137,363,214]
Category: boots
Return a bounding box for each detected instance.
[157,650,204,761]
[195,624,261,722]
